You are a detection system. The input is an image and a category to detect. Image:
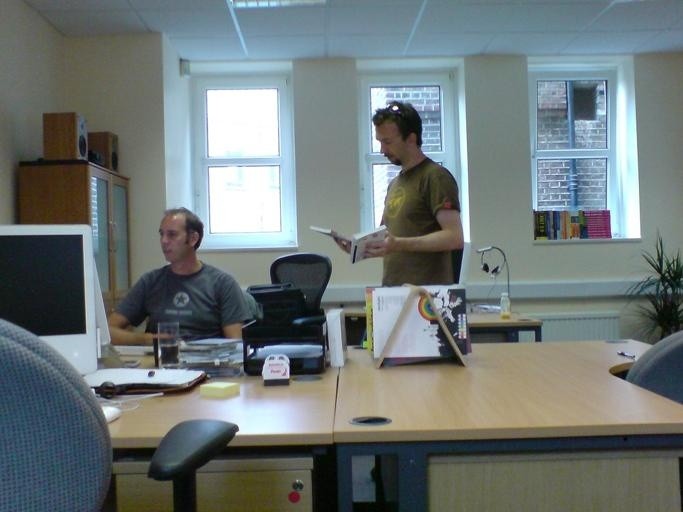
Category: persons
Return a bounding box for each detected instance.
[372,99,465,286]
[106,207,254,346]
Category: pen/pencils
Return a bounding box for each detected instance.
[616,350,636,359]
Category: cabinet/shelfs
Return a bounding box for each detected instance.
[16,160,130,318]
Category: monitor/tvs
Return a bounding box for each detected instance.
[1,224,98,375]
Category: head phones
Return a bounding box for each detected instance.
[480,247,506,276]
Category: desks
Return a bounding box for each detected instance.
[82,355,337,512]
[333,338,681,511]
[324,306,541,343]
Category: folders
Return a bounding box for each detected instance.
[82,367,208,400]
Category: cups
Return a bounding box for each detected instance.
[158,321,179,364]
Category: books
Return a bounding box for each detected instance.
[532,209,612,241]
[180,337,244,377]
[365,286,472,367]
[349,225,387,264]
[309,225,352,245]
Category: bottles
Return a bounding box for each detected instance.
[500,292,510,322]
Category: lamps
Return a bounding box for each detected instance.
[474,243,511,316]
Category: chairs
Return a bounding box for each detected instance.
[270,253,331,310]
[0,318,239,512]
[624,331,683,404]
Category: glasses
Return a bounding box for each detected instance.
[386,100,406,124]
